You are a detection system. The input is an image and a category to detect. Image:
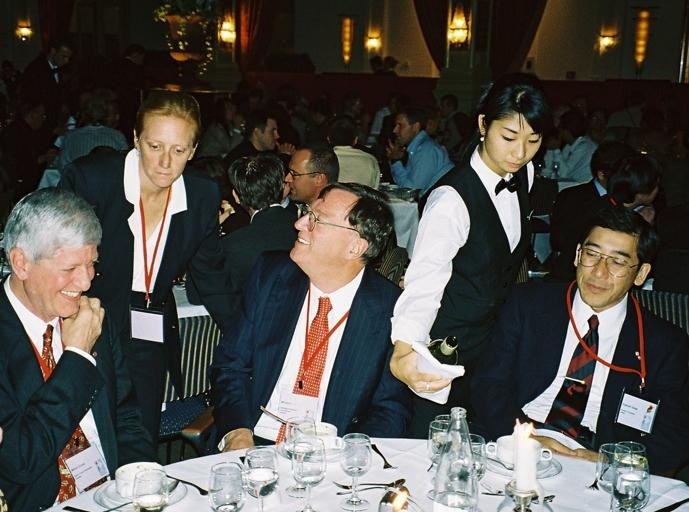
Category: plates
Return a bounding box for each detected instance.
[89,479,187,512]
[483,448,562,480]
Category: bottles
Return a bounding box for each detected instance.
[428,334,460,369]
[432,406,480,512]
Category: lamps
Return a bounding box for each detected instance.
[337,12,361,71]
[446,0,473,51]
[629,5,661,80]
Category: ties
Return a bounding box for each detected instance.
[546,315,600,441]
[293,297,332,397]
[40,324,107,503]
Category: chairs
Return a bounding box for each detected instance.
[36,170,689,470]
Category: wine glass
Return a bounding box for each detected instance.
[208,417,373,512]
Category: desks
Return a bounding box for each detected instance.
[36,170,689,512]
[36,437,688,512]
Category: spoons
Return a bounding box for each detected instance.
[104,479,179,512]
[336,479,405,496]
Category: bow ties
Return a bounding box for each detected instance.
[495,175,517,196]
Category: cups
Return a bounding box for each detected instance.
[524,438,552,465]
[427,413,487,490]
[131,470,169,512]
[113,461,166,499]
[486,435,515,465]
[594,439,652,512]
[381,182,399,192]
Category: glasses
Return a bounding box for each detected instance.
[287,168,320,178]
[302,207,363,232]
[577,244,639,278]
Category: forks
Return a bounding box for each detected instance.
[165,475,212,495]
[330,480,390,490]
[371,445,398,471]
